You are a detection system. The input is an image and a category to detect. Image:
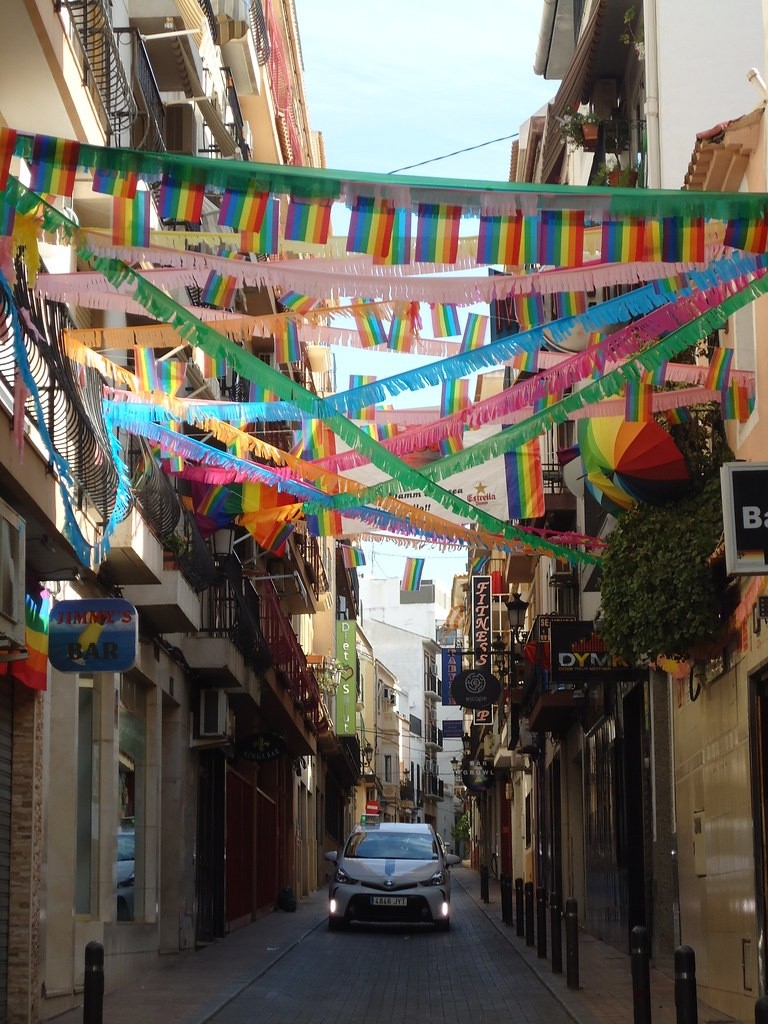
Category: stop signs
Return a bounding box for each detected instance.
[365,800,379,814]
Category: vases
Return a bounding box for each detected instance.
[581,124,600,147]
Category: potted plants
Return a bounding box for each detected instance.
[589,155,644,188]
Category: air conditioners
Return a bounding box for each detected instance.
[548,543,572,579]
[257,352,281,372]
[197,689,236,745]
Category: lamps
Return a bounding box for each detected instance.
[504,592,529,644]
[462,731,472,750]
[363,743,374,768]
[450,757,461,777]
[403,769,410,780]
[491,635,508,676]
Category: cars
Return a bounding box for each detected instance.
[116,831,135,922]
[323,821,461,932]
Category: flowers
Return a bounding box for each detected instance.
[553,102,604,155]
